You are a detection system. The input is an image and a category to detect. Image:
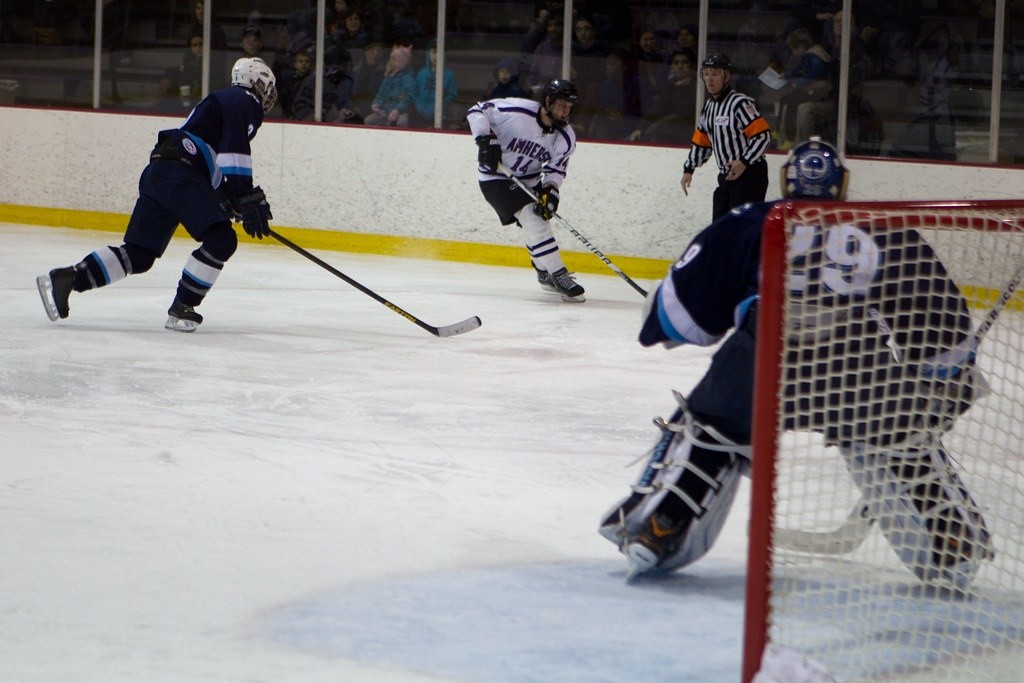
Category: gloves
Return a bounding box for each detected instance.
[231,186,274,240]
[532,186,560,221]
[475,134,503,175]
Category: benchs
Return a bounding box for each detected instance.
[0,1,1023,166]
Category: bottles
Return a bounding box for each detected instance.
[179,84,194,114]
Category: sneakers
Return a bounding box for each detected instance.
[935,532,980,599]
[550,267,586,303]
[620,507,684,585]
[165,296,203,333]
[532,259,559,294]
[37,266,76,322]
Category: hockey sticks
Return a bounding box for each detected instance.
[772,495,876,555]
[916,266,1023,381]
[269,229,482,337]
[498,162,647,297]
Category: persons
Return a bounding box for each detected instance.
[597,137,996,587]
[0,0,1024,163]
[681,55,773,223]
[36,57,278,332]
[467,80,587,302]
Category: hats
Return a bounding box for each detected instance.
[242,25,262,42]
[391,45,414,70]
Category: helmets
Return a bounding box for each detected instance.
[541,78,578,128]
[230,57,278,114]
[780,140,849,202]
[702,54,731,69]
